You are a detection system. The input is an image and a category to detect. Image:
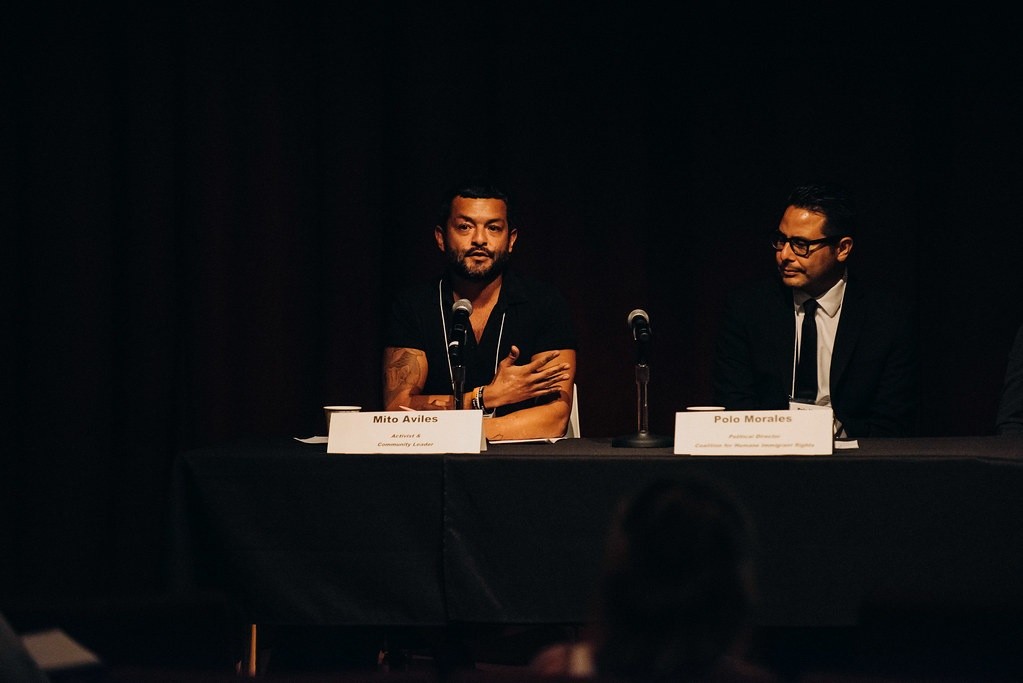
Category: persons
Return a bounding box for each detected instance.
[711,183,926,667]
[994,326,1023,435]
[344,172,581,667]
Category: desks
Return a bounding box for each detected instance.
[185,431,1023,628]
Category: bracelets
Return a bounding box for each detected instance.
[472,384,495,415]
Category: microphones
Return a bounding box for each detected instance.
[449,299,473,359]
[628,309,650,342]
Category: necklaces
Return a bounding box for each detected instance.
[439,279,506,390]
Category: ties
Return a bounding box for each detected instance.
[795,299,818,403]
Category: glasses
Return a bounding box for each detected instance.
[771,231,837,256]
